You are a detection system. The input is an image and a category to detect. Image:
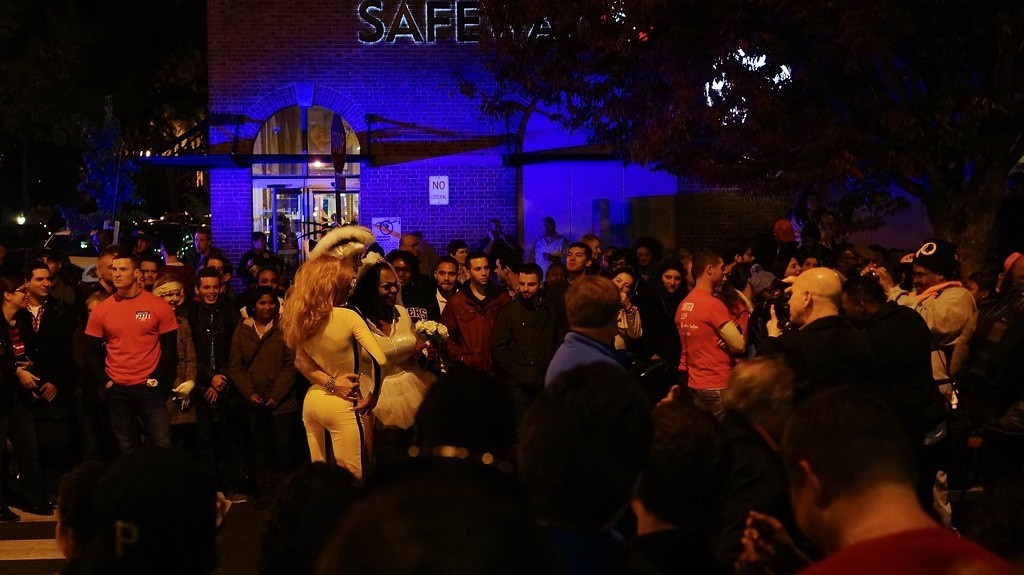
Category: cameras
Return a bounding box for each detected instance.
[762,291,791,318]
[871,267,886,279]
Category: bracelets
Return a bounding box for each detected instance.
[624,305,632,313]
[250,392,256,399]
[326,377,337,394]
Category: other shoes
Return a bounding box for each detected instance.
[224,490,248,503]
[27,498,55,512]
[0,505,20,523]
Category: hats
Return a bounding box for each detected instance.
[801,221,821,240]
[81,451,215,575]
[774,218,797,242]
[914,236,958,275]
[150,274,186,306]
[751,265,791,296]
[132,230,150,241]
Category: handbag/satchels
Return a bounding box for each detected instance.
[914,409,966,464]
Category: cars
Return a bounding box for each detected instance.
[38,229,104,292]
[145,222,201,264]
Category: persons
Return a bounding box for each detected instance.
[0,215,1024,575]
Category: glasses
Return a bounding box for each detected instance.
[14,288,28,294]
